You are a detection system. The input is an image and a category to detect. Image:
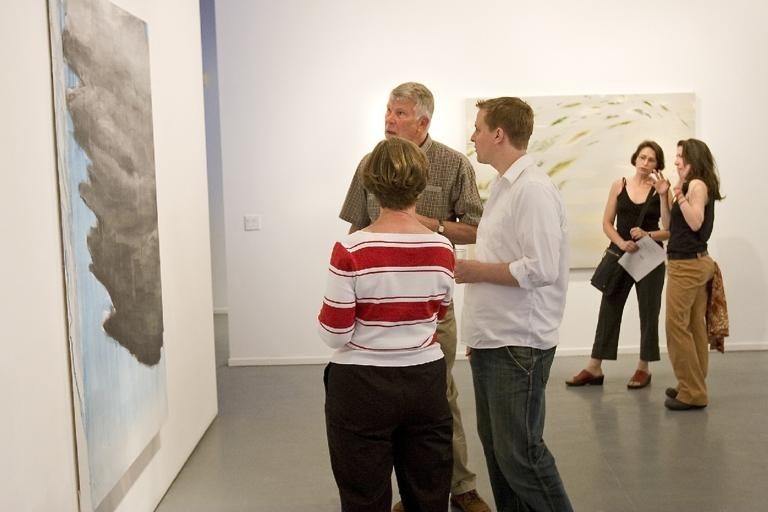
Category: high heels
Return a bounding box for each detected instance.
[564,369,605,387]
[627,370,653,389]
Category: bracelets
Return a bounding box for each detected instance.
[674,192,687,206]
[648,232,651,237]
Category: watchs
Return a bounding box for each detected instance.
[438,219,445,234]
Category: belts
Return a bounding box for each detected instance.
[668,250,708,261]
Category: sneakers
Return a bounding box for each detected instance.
[665,386,680,398]
[450,488,491,512]
[664,398,707,410]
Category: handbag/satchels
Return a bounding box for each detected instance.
[591,247,623,296]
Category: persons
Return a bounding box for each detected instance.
[451,97,574,510]
[339,82,493,512]
[312,136,456,512]
[646,138,728,409]
[565,140,673,390]
